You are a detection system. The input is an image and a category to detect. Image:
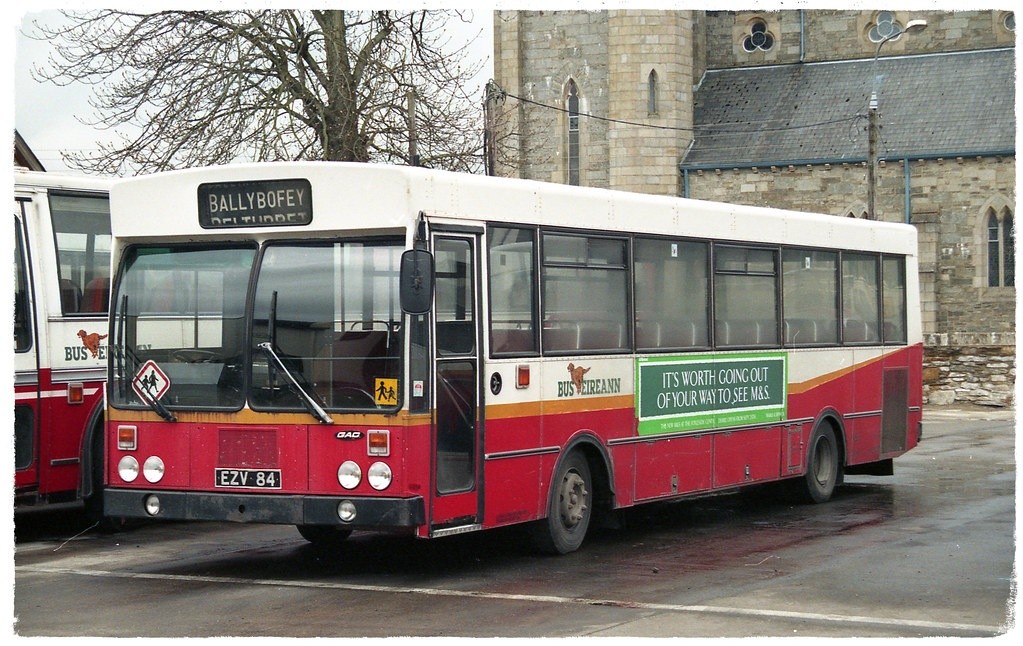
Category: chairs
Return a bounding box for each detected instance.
[60,275,110,315]
[491,319,882,354]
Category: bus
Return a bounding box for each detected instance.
[14,166,156,535]
[102,158,924,558]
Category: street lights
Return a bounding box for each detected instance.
[867,18,928,218]
